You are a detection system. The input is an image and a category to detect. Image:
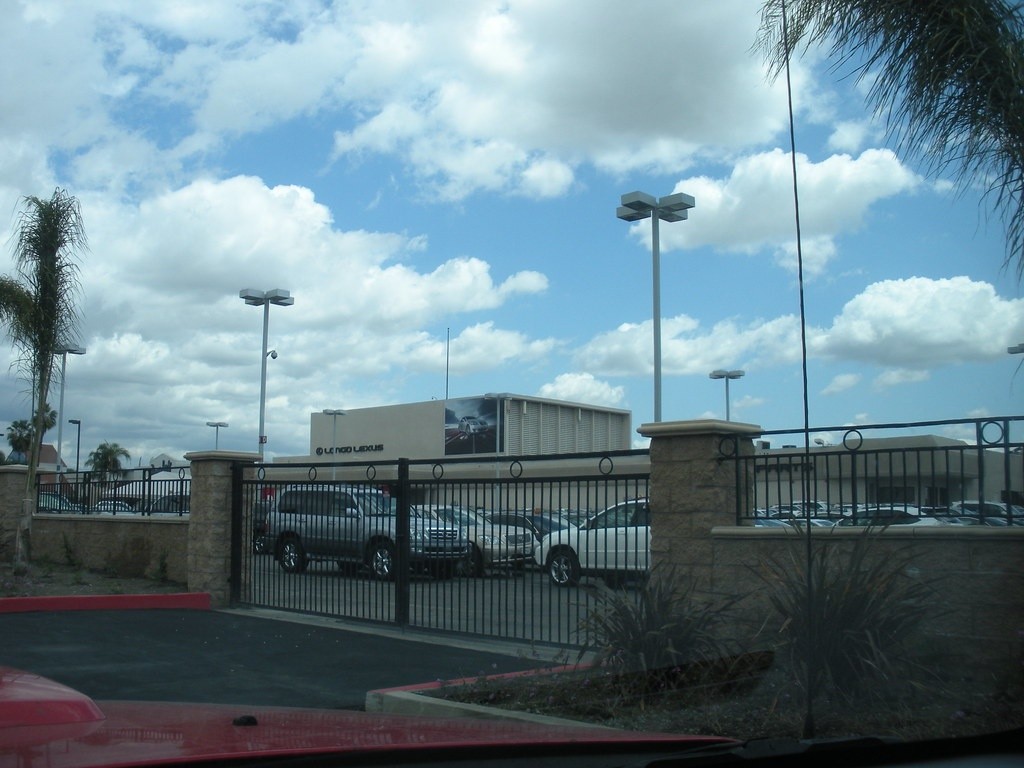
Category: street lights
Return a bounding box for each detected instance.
[51,342,86,497]
[205,421,229,450]
[237,287,295,464]
[708,369,745,422]
[615,190,698,424]
[322,407,346,479]
[68,418,82,502]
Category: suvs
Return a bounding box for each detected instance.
[265,482,473,580]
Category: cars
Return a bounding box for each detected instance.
[390,503,600,576]
[751,500,1024,530]
[457,415,488,436]
[38,491,271,554]
[532,494,654,588]
